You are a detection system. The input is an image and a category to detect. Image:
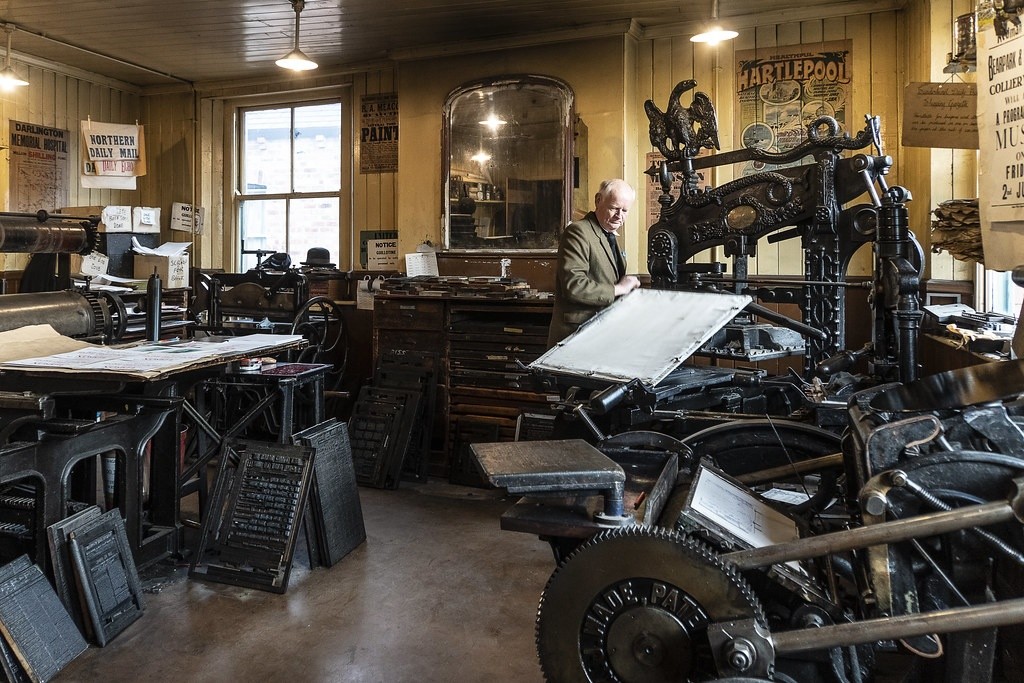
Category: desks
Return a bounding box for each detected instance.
[921,318,1016,376]
[1,335,334,562]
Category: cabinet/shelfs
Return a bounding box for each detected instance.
[109,286,192,341]
[372,294,560,489]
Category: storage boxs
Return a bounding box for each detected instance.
[61,206,189,290]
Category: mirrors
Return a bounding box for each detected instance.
[442,73,574,259]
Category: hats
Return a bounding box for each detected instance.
[300,247,336,266]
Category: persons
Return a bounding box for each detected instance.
[547,179,641,400]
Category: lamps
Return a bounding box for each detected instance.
[689,0,739,46]
[275,0,318,71]
[0,23,29,92]
[470,89,507,162]
